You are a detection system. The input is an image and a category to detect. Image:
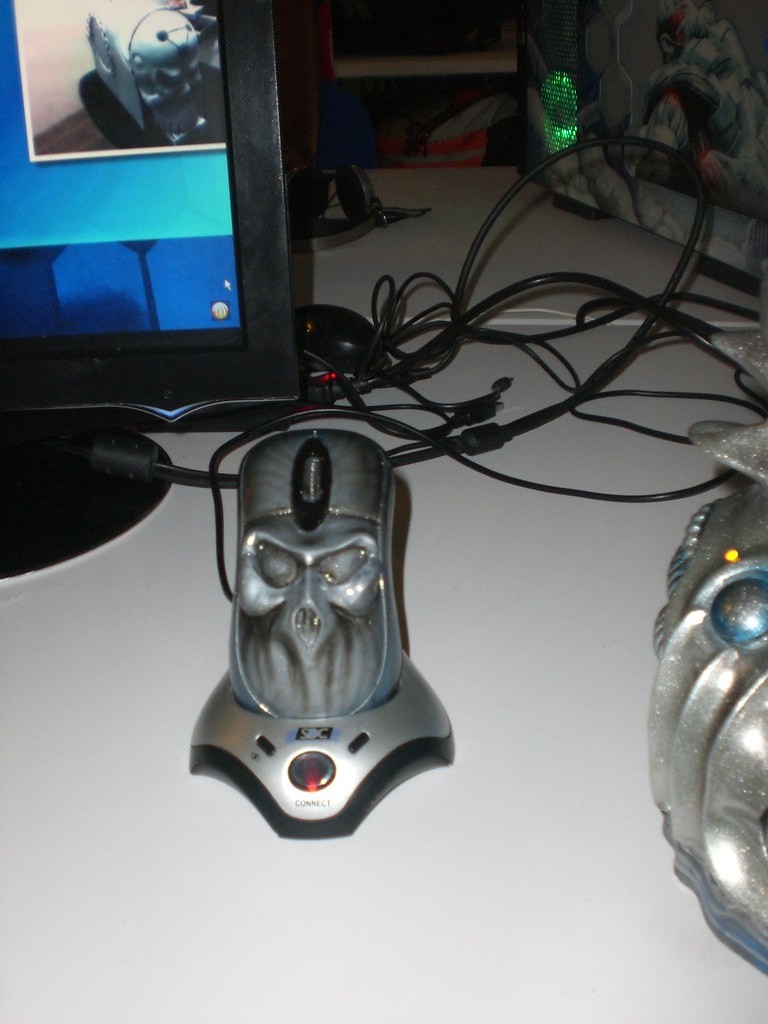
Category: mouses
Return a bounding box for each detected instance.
[228,428,402,719]
[292,304,378,375]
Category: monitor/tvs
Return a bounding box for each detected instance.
[0,0,306,580]
